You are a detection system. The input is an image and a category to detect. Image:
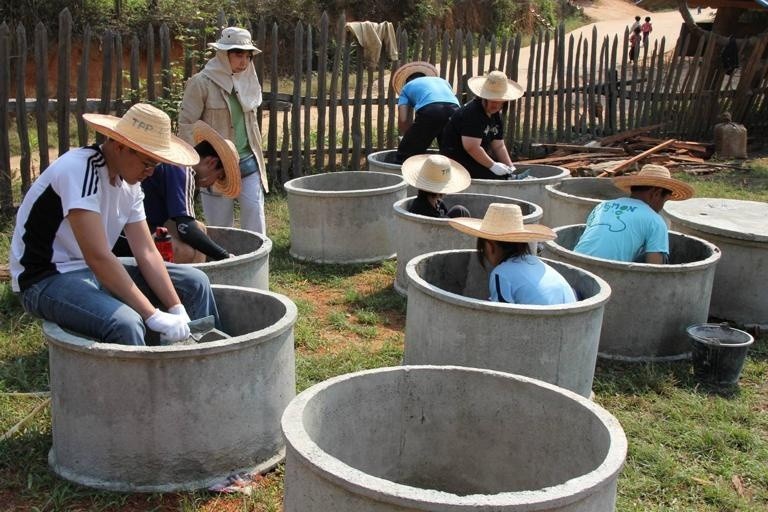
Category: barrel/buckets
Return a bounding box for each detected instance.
[685,321,754,389]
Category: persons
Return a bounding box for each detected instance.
[572,164,695,264]
[178,27,269,235]
[401,154,472,219]
[112,117,242,263]
[449,202,578,305]
[391,61,461,165]
[9,104,222,346]
[640,17,652,47]
[437,69,525,180]
[627,26,642,64]
[629,17,642,35]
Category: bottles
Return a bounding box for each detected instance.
[151,226,176,264]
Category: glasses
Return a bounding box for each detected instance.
[129,150,162,168]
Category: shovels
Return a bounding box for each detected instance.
[511,167,530,175]
[187,315,215,333]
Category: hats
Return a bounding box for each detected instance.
[206,27,262,56]
[447,203,558,243]
[467,70,525,102]
[392,61,440,97]
[401,153,472,194]
[82,102,200,167]
[193,120,242,200]
[610,164,695,201]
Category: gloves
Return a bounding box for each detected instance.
[146,303,193,342]
[489,162,513,176]
